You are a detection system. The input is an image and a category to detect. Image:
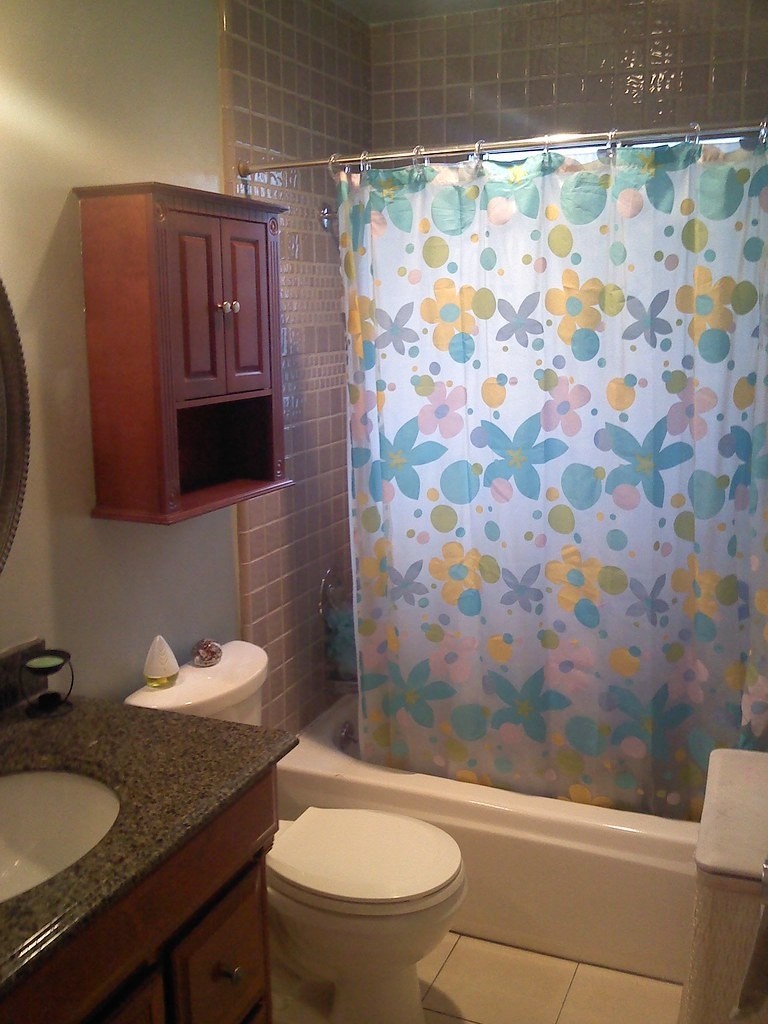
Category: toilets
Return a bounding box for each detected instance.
[127,639,469,1024]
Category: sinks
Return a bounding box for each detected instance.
[0,769,119,902]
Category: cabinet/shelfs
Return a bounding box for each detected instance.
[1,764,278,1024]
[72,182,295,526]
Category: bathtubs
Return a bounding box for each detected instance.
[272,693,700,986]
